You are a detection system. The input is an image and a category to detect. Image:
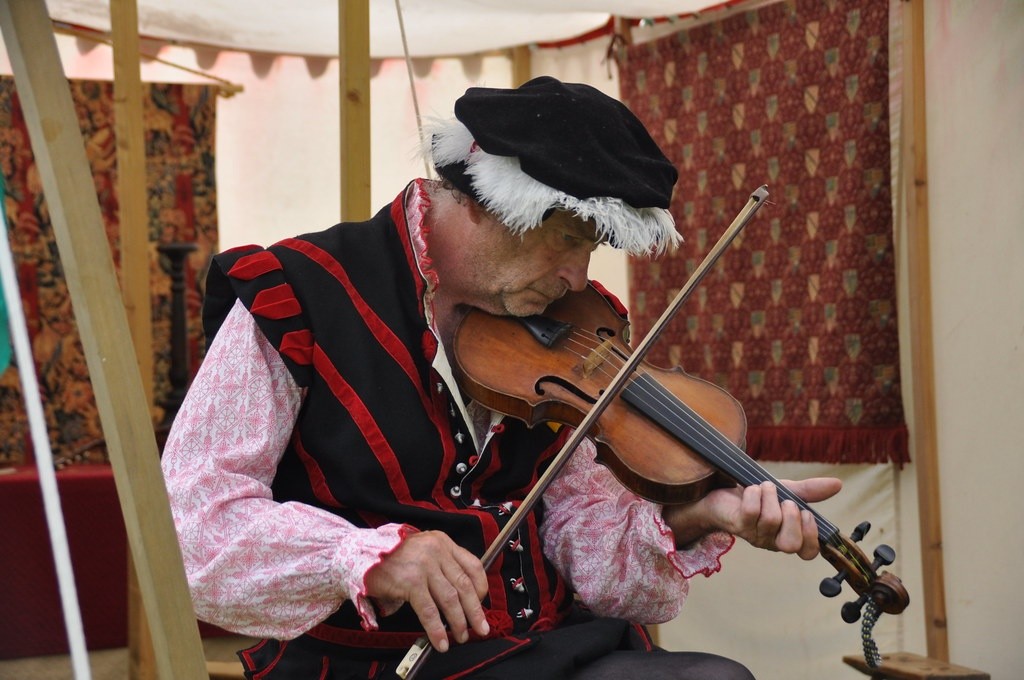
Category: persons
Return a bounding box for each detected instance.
[160,76,843,680]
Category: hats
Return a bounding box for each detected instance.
[435,75,684,260]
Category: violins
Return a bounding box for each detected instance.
[451,278,914,625]
